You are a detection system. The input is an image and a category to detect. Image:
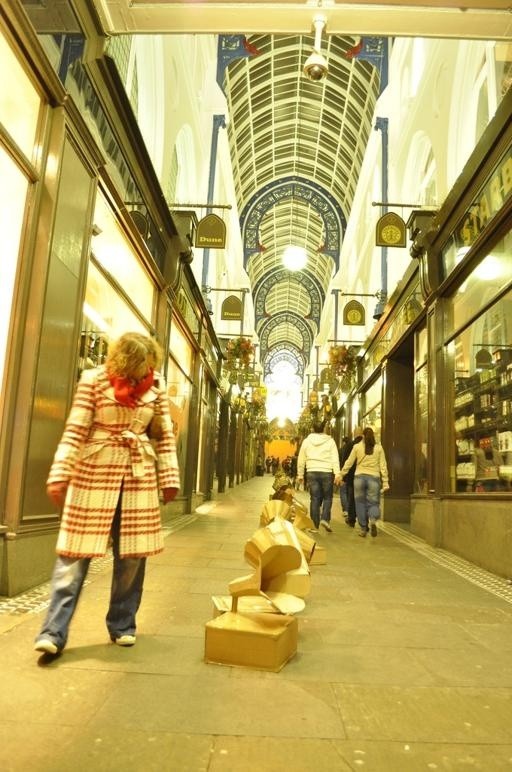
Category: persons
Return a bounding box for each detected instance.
[466,428,508,492]
[256,420,390,538]
[33,332,180,654]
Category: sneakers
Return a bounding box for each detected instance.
[358,522,377,538]
[116,635,137,646]
[345,518,355,529]
[320,519,333,534]
[34,640,63,655]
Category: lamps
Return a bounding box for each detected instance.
[302,15,330,83]
[281,195,308,273]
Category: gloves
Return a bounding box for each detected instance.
[160,486,179,506]
[47,481,67,505]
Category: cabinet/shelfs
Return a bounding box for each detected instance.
[454,367,512,493]
